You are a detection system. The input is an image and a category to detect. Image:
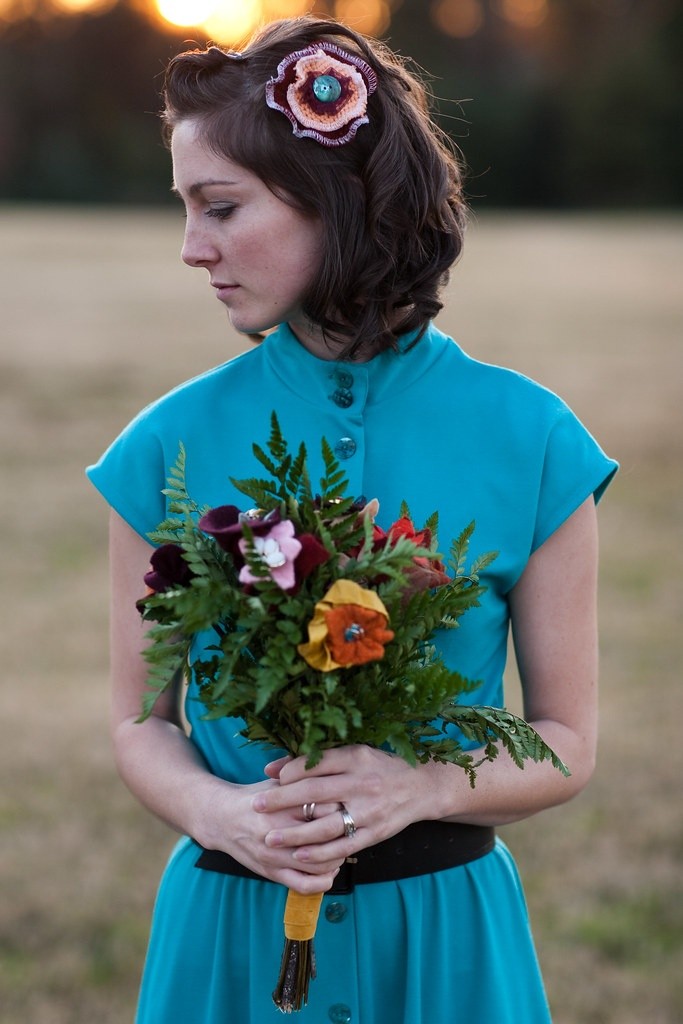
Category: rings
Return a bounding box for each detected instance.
[339,810,357,839]
[338,802,346,811]
[303,803,315,820]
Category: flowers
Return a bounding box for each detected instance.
[136,414,573,1011]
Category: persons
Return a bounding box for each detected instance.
[85,18,619,1023]
